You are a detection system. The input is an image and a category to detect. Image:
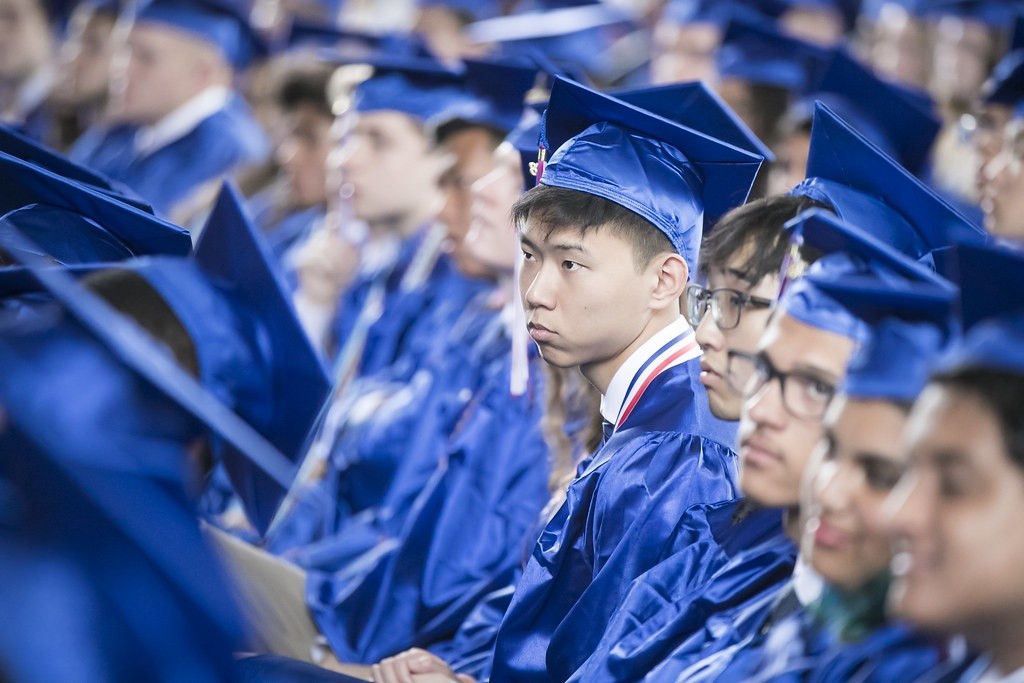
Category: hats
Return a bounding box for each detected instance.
[783,207,957,338]
[930,237,1024,377]
[805,274,955,401]
[0,123,340,682]
[613,79,771,162]
[653,0,1023,165]
[789,100,995,260]
[128,0,596,150]
[536,73,763,283]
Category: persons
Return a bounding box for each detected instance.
[0,0,1024,683]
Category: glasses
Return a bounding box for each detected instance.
[725,349,834,421]
[686,282,773,331]
